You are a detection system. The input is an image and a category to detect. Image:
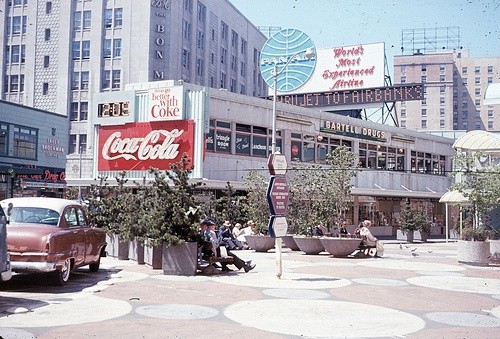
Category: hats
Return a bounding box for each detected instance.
[200,219,207,225]
[223,221,231,226]
[207,220,214,226]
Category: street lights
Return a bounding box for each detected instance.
[78,143,91,203]
[270,50,314,277]
[8,167,15,199]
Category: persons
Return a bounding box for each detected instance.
[200,220,256,272]
[313,225,324,236]
[360,220,384,258]
[340,221,364,238]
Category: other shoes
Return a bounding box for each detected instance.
[245,246,249,250]
[246,260,252,264]
[233,246,236,250]
[239,245,243,250]
[222,267,233,271]
[374,254,382,258]
[213,264,222,268]
[245,264,256,272]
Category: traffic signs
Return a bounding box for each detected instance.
[267,153,290,238]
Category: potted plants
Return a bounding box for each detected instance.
[91,153,212,275]
[448,147,500,264]
[246,145,363,257]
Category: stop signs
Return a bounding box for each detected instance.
[292,145,299,156]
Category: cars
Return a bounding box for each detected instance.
[0,197,109,285]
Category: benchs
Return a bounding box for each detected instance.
[196,242,233,272]
[338,234,377,256]
